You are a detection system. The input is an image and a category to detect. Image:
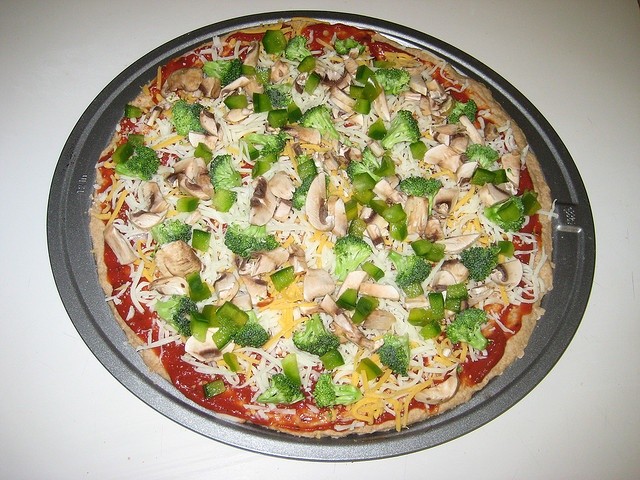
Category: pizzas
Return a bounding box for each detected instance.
[88,17,553,437]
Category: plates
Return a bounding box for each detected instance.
[46,10,595,462]
